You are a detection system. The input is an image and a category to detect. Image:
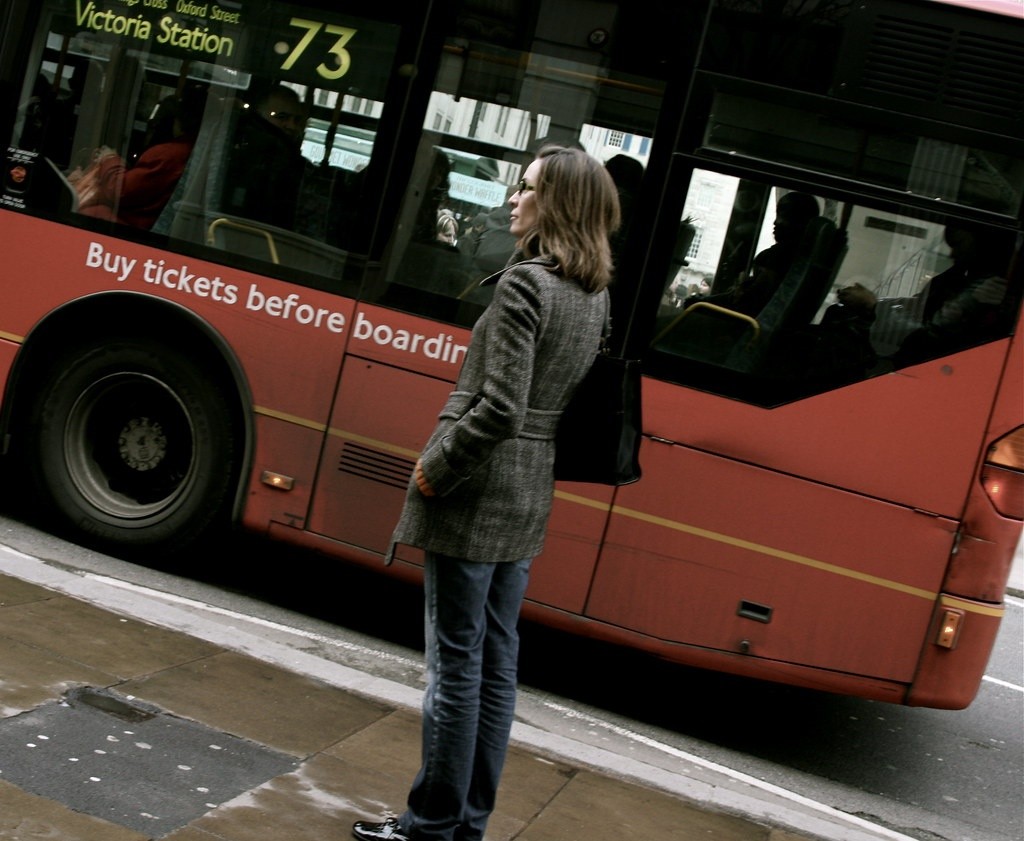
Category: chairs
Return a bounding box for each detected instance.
[193,108,367,281]
[664,221,695,292]
[724,216,848,377]
[297,166,353,240]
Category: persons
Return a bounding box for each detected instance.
[15,44,1013,365]
[348,147,618,841]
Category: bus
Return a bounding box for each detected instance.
[0,0,1024,711]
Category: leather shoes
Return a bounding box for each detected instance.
[352,817,411,841]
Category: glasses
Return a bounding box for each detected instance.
[519,178,537,195]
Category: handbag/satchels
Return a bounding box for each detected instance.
[553,286,643,486]
